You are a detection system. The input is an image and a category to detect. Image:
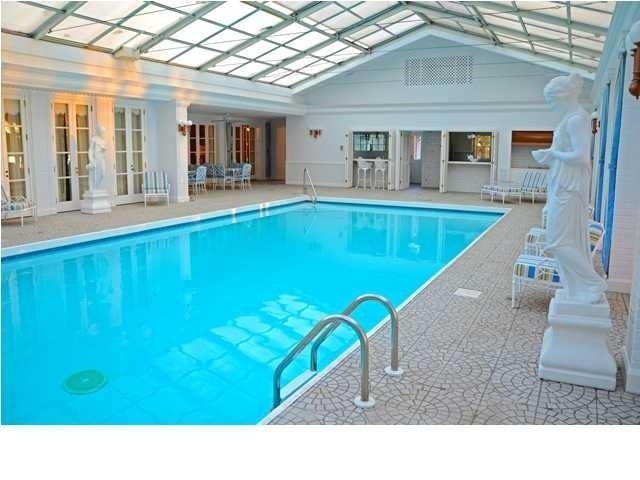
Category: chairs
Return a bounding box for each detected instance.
[141,169,171,209]
[355,155,387,193]
[188,160,255,198]
[510,199,605,310]
[479,163,549,206]
[1,184,38,228]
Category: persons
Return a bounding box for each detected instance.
[531,77,608,300]
